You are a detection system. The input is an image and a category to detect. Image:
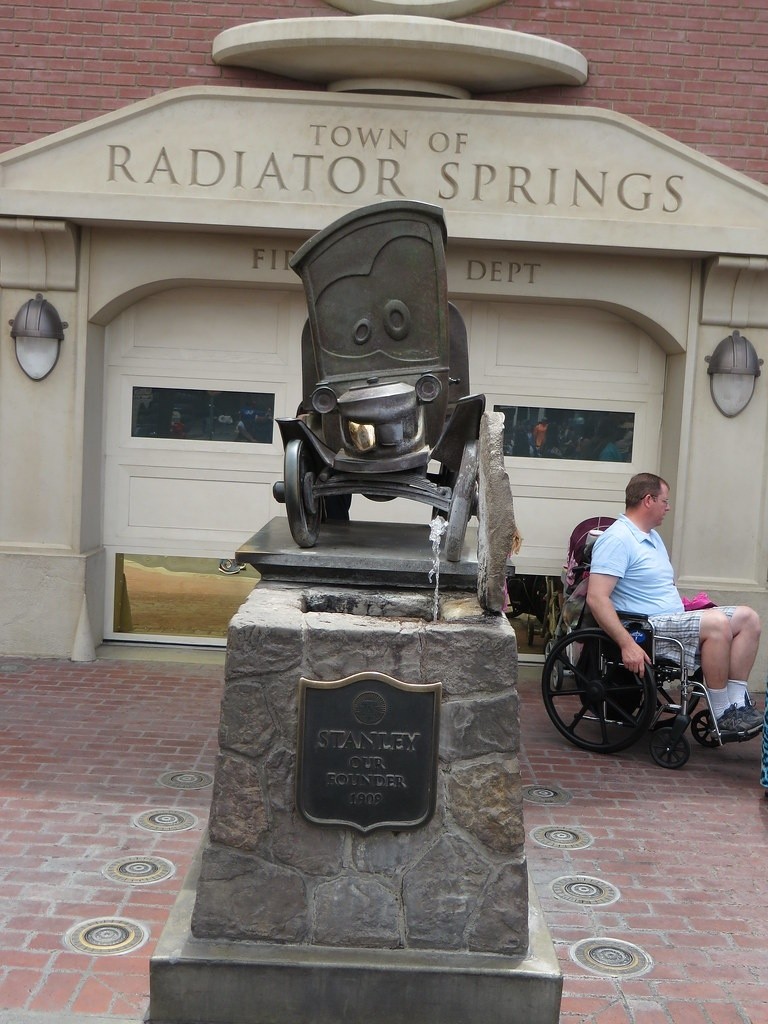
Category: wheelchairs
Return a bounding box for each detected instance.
[504,574,567,647]
[539,562,763,770]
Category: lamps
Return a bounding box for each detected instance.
[9,292,70,380]
[704,328,764,418]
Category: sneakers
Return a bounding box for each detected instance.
[737,704,764,718]
[710,703,763,741]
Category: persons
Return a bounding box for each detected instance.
[584,472,765,737]
[510,416,633,462]
